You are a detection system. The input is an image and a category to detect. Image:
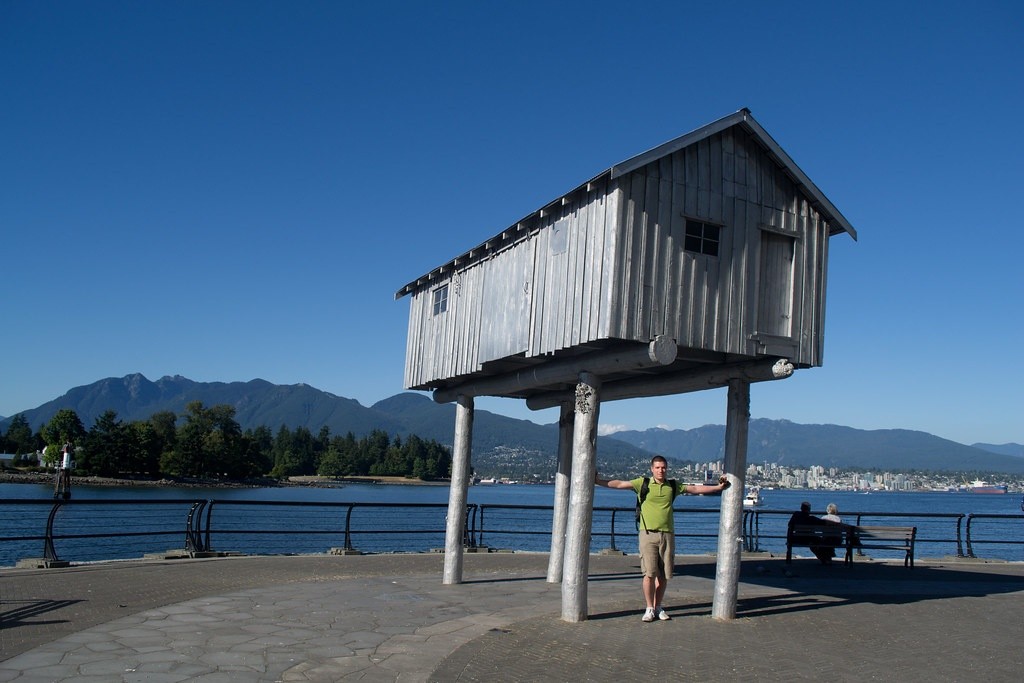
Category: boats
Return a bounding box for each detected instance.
[742,487,765,507]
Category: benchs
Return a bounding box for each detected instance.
[786,524,916,568]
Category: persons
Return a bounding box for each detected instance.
[788,502,843,560]
[595,456,727,622]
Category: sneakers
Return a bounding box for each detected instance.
[642,608,655,621]
[655,609,670,621]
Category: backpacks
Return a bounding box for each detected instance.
[635,477,676,522]
